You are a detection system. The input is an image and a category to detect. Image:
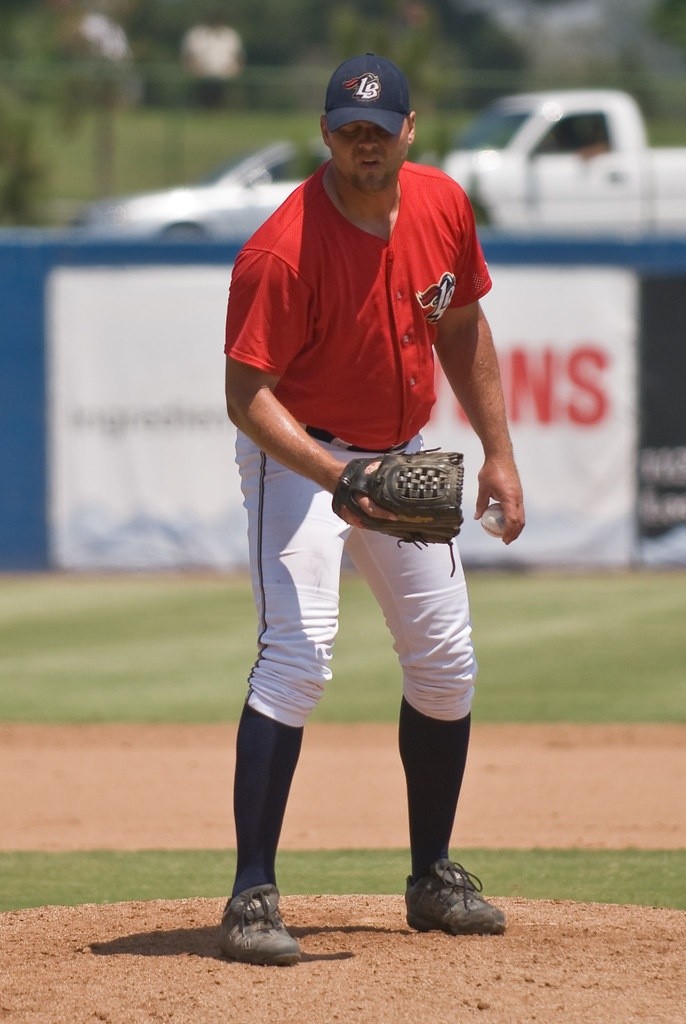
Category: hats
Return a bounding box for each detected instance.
[325,53,409,135]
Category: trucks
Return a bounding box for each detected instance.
[434,86,686,241]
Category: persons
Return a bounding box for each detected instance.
[552,116,609,160]
[66,2,241,111]
[224,52,525,965]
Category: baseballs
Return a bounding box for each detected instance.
[478,501,511,541]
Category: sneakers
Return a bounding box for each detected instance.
[405,857,505,936]
[221,883,301,966]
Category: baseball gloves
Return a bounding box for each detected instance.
[329,446,468,547]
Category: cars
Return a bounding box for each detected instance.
[60,135,334,245]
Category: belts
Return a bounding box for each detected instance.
[306,425,409,453]
